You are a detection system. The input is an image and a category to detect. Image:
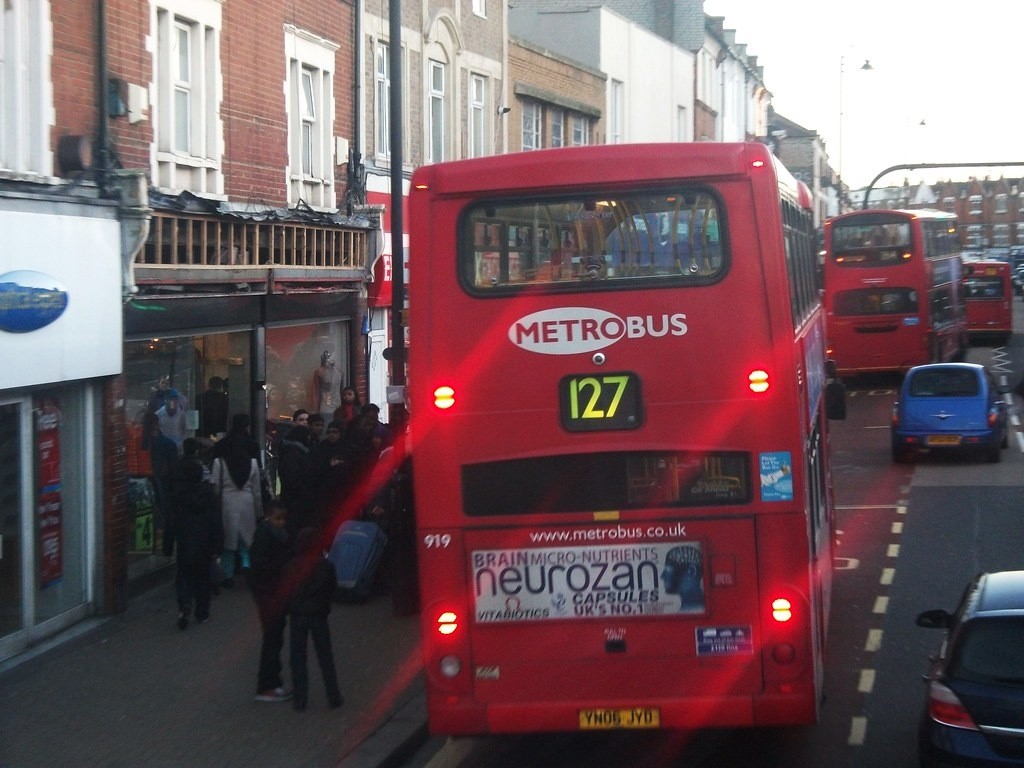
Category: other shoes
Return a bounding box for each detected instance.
[177,603,211,631]
[256,687,294,702]
[220,578,232,588]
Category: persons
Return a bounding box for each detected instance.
[162,462,227,629]
[283,527,346,710]
[242,507,294,701]
[310,351,348,427]
[252,387,393,557]
[146,373,260,555]
[212,431,264,584]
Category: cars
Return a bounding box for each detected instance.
[891,362,1012,464]
[984,246,1024,295]
[915,569,1024,768]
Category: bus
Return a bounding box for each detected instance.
[962,260,1012,340]
[824,209,974,383]
[407,140,846,740]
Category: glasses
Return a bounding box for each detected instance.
[326,430,340,435]
